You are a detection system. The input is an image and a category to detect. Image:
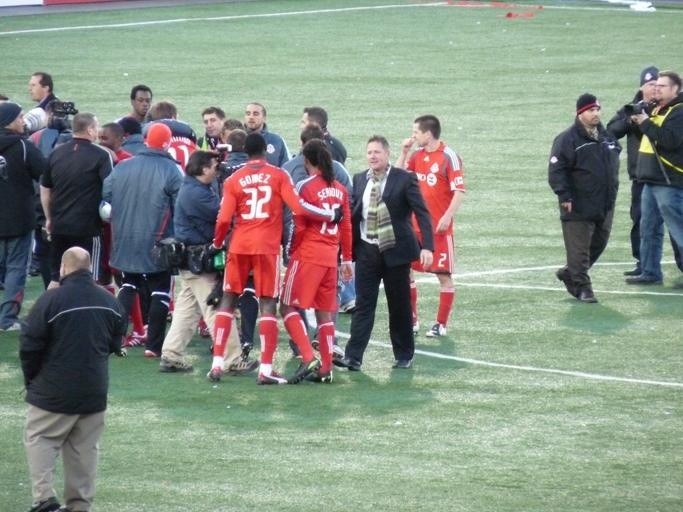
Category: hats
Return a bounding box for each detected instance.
[577,93,601,115]
[639,66,660,88]
[146,123,172,151]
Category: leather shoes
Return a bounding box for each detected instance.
[625,273,662,284]
[0,103,22,129]
[577,289,598,303]
[555,266,579,298]
[332,357,361,371]
[391,356,414,369]
[624,267,642,275]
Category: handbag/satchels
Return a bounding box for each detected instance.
[150,239,186,267]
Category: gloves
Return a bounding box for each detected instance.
[329,204,344,224]
[201,243,227,266]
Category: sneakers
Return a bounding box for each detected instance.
[121,299,356,358]
[256,371,288,385]
[207,365,222,383]
[159,357,193,372]
[288,357,320,384]
[0,322,21,332]
[426,320,447,337]
[29,251,41,276]
[24,496,61,512]
[305,371,332,384]
[52,504,88,512]
[229,359,259,376]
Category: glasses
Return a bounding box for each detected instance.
[654,84,670,89]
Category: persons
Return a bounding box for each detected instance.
[548,92,624,303]
[623,70,683,285]
[606,66,682,276]
[0,74,112,333]
[98,84,358,386]
[394,115,466,338]
[20,246,128,512]
[332,135,436,371]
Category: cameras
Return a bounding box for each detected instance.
[213,144,246,184]
[624,99,660,118]
